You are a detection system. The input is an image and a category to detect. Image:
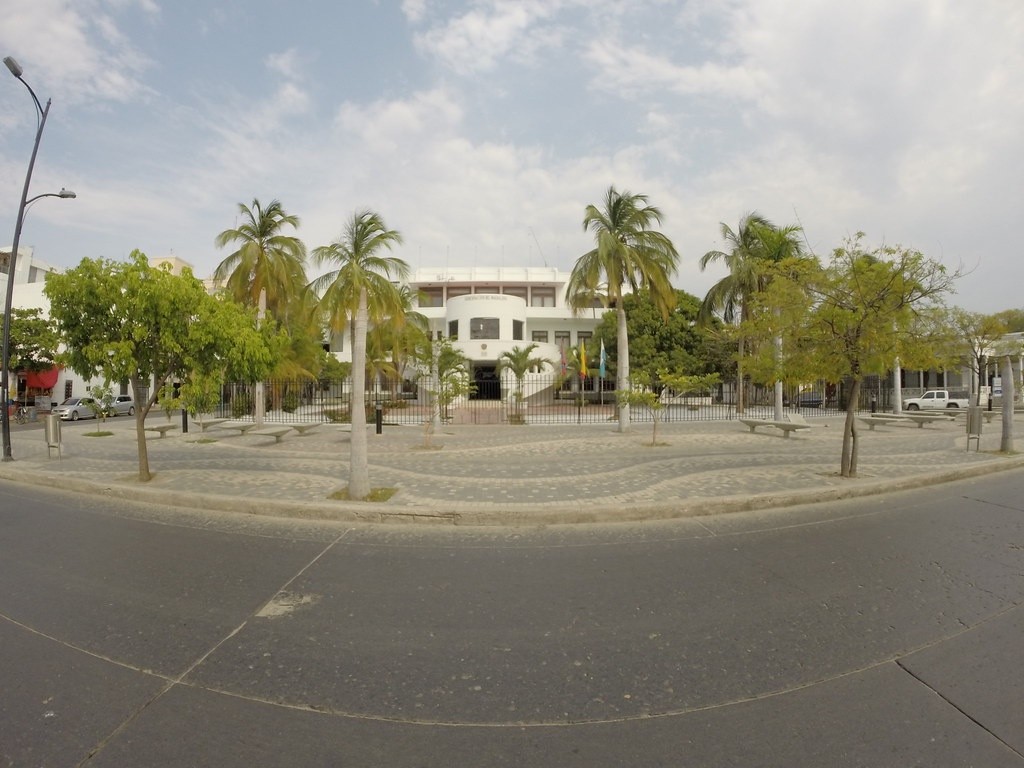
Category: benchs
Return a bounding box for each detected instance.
[250,426,292,442]
[740,419,812,439]
[217,421,256,435]
[128,422,177,438]
[288,421,324,436]
[856,409,1002,430]
[191,418,230,433]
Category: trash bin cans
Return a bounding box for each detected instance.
[966,407,982,434]
[28,406,38,421]
[44,415,62,443]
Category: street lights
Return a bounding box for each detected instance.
[1,55,78,463]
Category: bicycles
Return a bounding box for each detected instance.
[13,403,27,425]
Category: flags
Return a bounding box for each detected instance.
[560,340,567,375]
[599,343,606,378]
[579,343,586,379]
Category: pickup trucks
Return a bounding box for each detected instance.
[903,390,970,418]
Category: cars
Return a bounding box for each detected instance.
[51,397,101,421]
[993,389,1002,398]
[99,395,136,417]
